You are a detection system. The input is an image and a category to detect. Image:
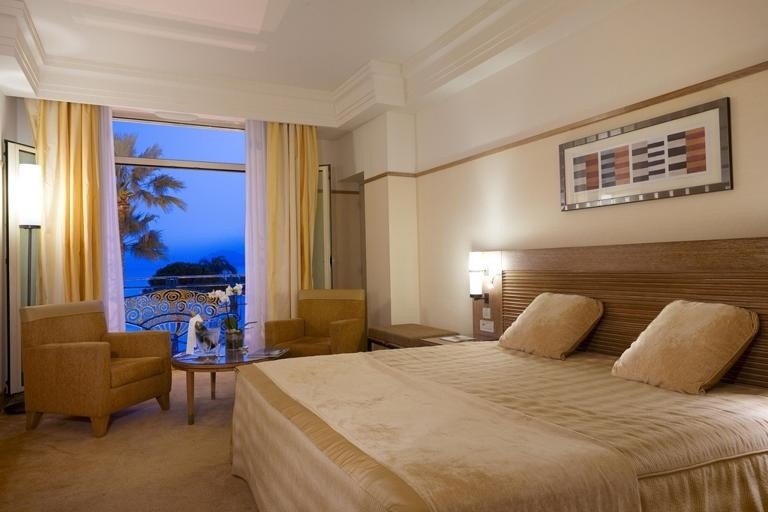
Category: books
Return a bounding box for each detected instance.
[440,335,477,343]
[246,348,290,358]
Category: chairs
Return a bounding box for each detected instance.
[261,287,367,356]
[17,298,174,439]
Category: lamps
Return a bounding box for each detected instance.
[15,161,44,231]
[467,267,490,304]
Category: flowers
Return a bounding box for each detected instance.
[208,283,244,328]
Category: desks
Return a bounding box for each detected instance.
[368,322,460,351]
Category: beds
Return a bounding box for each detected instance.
[228,236,768,512]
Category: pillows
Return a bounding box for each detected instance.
[610,298,761,398]
[498,290,605,362]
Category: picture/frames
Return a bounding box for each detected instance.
[558,96,735,212]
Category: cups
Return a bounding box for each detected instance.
[196,319,221,354]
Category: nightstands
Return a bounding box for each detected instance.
[420,332,498,346]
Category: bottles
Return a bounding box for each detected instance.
[190,311,215,350]
